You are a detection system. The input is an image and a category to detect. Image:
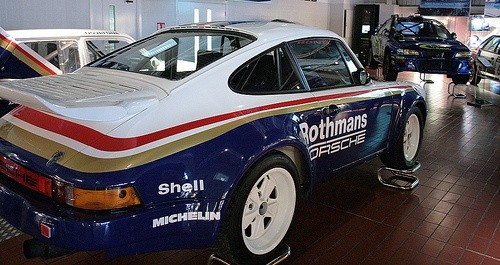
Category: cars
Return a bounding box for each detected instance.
[473,35,500,84]
[0,19,426,265]
[6,31,197,74]
[368,14,473,86]
